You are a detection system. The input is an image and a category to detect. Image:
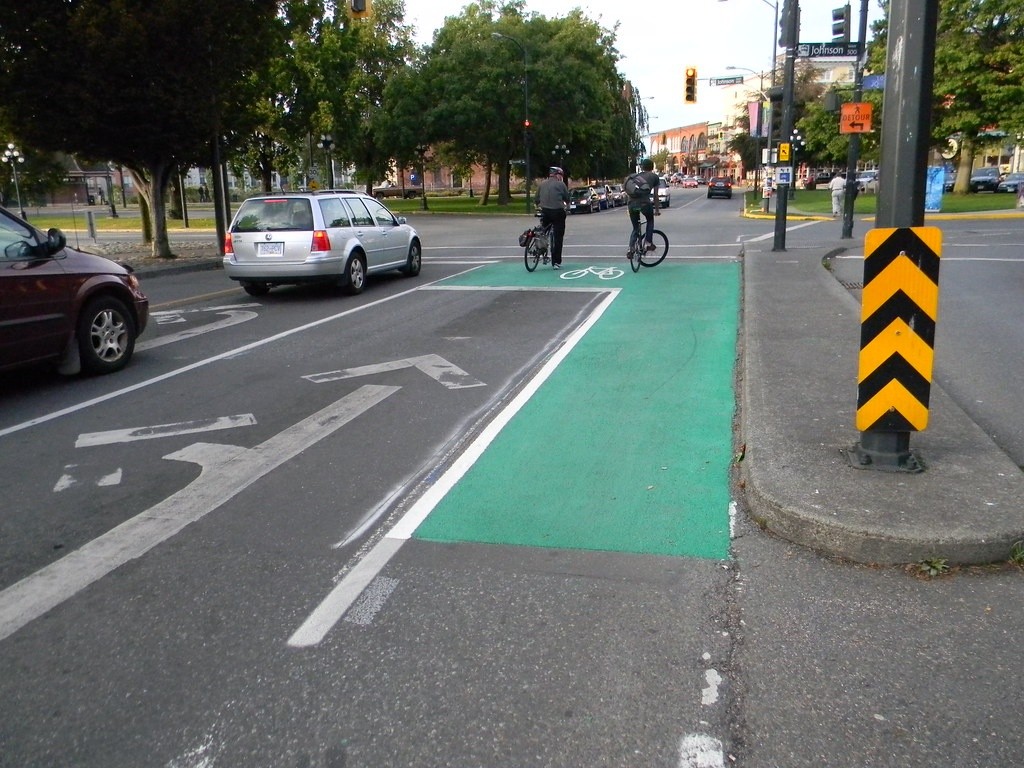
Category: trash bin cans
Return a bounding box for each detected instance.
[88,195,95,206]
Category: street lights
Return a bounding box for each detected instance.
[726,66,764,99]
[1,143,28,220]
[491,32,532,213]
[790,129,806,186]
[551,138,570,169]
[717,0,779,213]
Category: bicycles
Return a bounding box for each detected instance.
[524,208,571,272]
[627,207,669,273]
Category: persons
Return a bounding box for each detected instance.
[627,159,660,258]
[98,188,105,204]
[830,172,845,217]
[737,175,740,187]
[535,167,570,270]
[198,186,210,203]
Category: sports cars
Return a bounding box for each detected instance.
[372,181,425,200]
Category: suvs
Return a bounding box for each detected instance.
[222,186,423,298]
[970,167,1001,193]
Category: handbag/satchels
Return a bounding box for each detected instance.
[536,234,548,254]
[622,173,651,198]
[519,228,534,246]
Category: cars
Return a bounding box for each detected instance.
[945,173,957,191]
[588,184,615,210]
[656,172,707,189]
[814,170,879,190]
[563,186,601,213]
[0,205,149,379]
[997,173,1024,193]
[609,185,630,207]
[649,177,671,209]
[706,177,732,198]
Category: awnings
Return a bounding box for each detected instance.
[699,162,716,167]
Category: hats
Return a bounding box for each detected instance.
[642,159,653,170]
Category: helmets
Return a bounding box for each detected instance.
[550,166,564,175]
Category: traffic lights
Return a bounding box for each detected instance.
[831,5,851,42]
[684,64,699,104]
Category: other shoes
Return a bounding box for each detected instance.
[553,263,563,269]
[627,252,634,259]
[647,244,656,250]
